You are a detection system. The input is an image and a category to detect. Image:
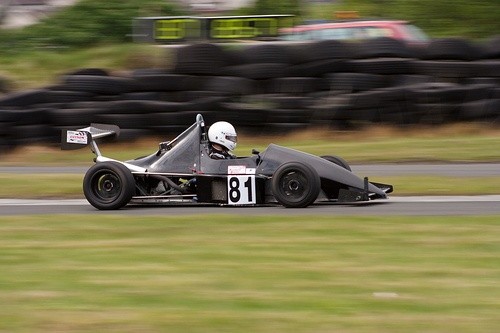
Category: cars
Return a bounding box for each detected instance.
[280,19,433,54]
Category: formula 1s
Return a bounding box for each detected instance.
[61,114,394,211]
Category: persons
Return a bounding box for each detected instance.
[207,120,239,159]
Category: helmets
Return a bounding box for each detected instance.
[208,121,237,151]
